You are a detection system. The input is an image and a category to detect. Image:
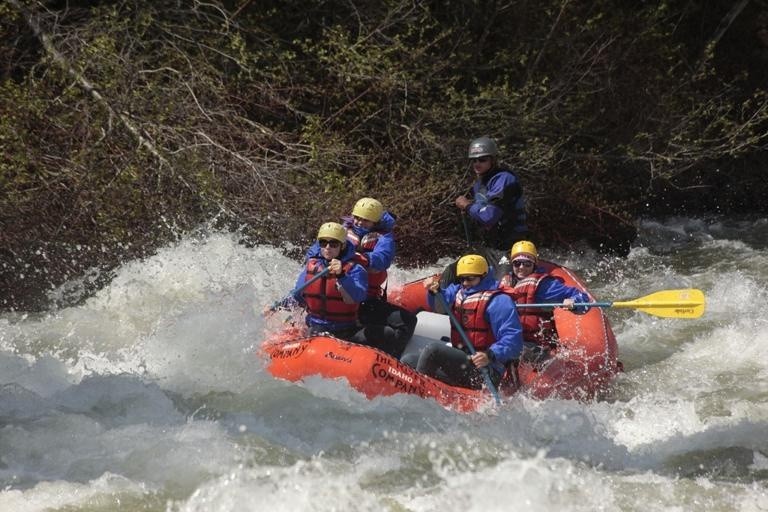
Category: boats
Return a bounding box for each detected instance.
[256,257,625,415]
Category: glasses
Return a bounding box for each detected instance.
[319,238,340,248]
[460,275,480,281]
[472,157,491,163]
[513,261,532,268]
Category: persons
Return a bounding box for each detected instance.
[439,134,529,290]
[261,222,368,343]
[497,238,592,368]
[397,253,524,390]
[303,196,418,360]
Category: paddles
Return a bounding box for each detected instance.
[515,289,705,318]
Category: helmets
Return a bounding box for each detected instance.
[468,136,498,158]
[353,197,383,222]
[317,222,347,244]
[510,240,540,265]
[456,255,488,277]
